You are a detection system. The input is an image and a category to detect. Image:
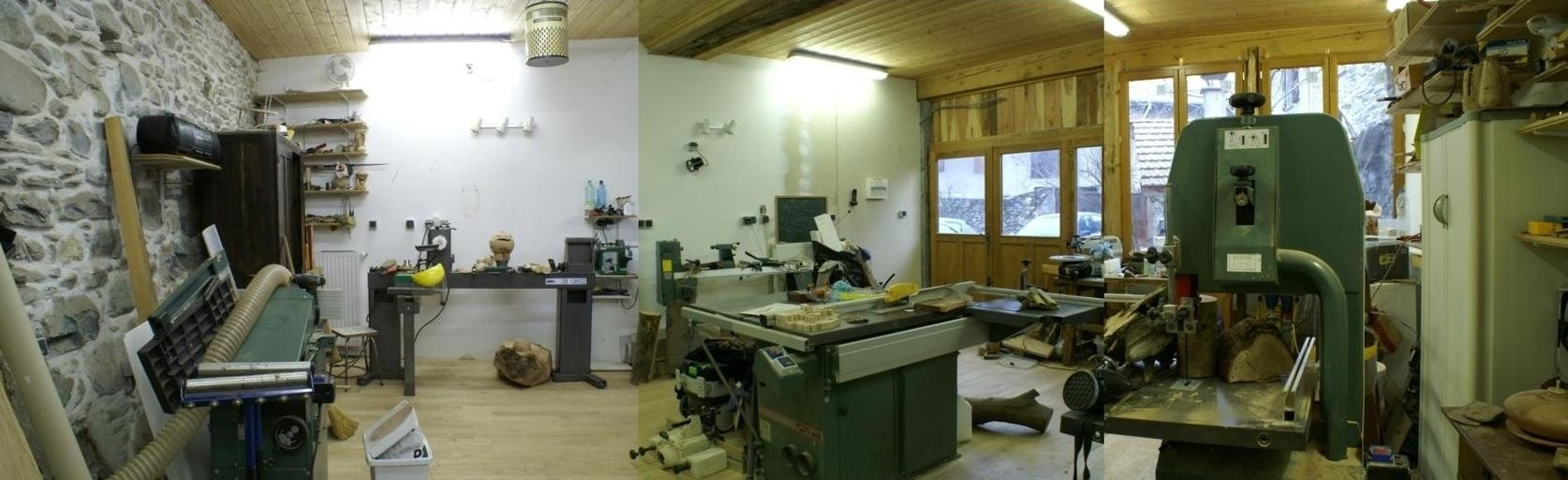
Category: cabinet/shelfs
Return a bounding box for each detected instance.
[199,130,305,290]
[584,208,639,301]
[1381,0,1568,480]
[256,88,372,228]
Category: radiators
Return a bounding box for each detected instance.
[310,247,369,349]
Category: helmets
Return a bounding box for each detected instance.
[410,258,447,287]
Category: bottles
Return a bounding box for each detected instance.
[1478,59,1502,108]
[584,179,594,211]
[1396,189,1412,236]
[595,180,605,209]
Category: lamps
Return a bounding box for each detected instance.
[1102,7,1131,39]
[698,115,741,139]
[522,0,570,68]
[789,50,890,82]
[470,114,538,135]
[686,140,709,172]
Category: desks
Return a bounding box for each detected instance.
[1051,275,1105,368]
[1103,272,1248,336]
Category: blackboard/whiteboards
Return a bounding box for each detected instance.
[774,195,827,244]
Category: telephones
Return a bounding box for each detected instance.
[850,189,858,205]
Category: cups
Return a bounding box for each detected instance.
[637,219,651,227]
[1364,207,1379,241]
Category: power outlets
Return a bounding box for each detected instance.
[759,214,769,226]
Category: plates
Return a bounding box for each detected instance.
[816,295,886,313]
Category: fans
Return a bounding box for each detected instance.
[324,52,358,89]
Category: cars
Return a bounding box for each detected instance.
[1007,212,1140,254]
[937,214,979,237]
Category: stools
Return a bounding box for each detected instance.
[328,324,383,394]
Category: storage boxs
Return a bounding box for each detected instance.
[1365,236,1411,285]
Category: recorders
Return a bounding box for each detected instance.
[137,115,221,164]
